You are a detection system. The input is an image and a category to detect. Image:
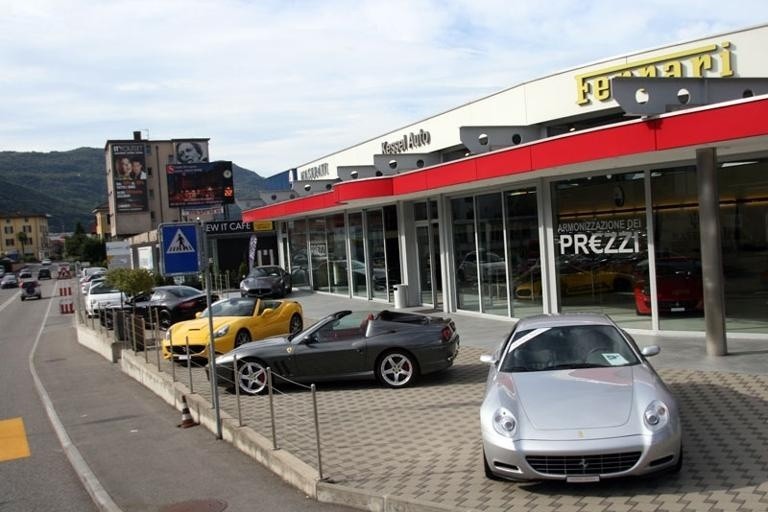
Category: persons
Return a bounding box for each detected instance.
[177,142,209,164]
[115,157,132,180]
[132,161,145,179]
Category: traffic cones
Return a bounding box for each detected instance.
[177,394,199,428]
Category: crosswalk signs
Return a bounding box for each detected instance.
[158,222,202,277]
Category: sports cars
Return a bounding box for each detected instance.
[479,313,683,483]
[512,256,715,316]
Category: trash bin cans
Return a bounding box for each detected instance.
[393,284,407,308]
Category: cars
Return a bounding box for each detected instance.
[307,258,388,287]
[239,264,293,297]
[77,263,129,320]
[0,257,52,302]
[456,249,509,284]
